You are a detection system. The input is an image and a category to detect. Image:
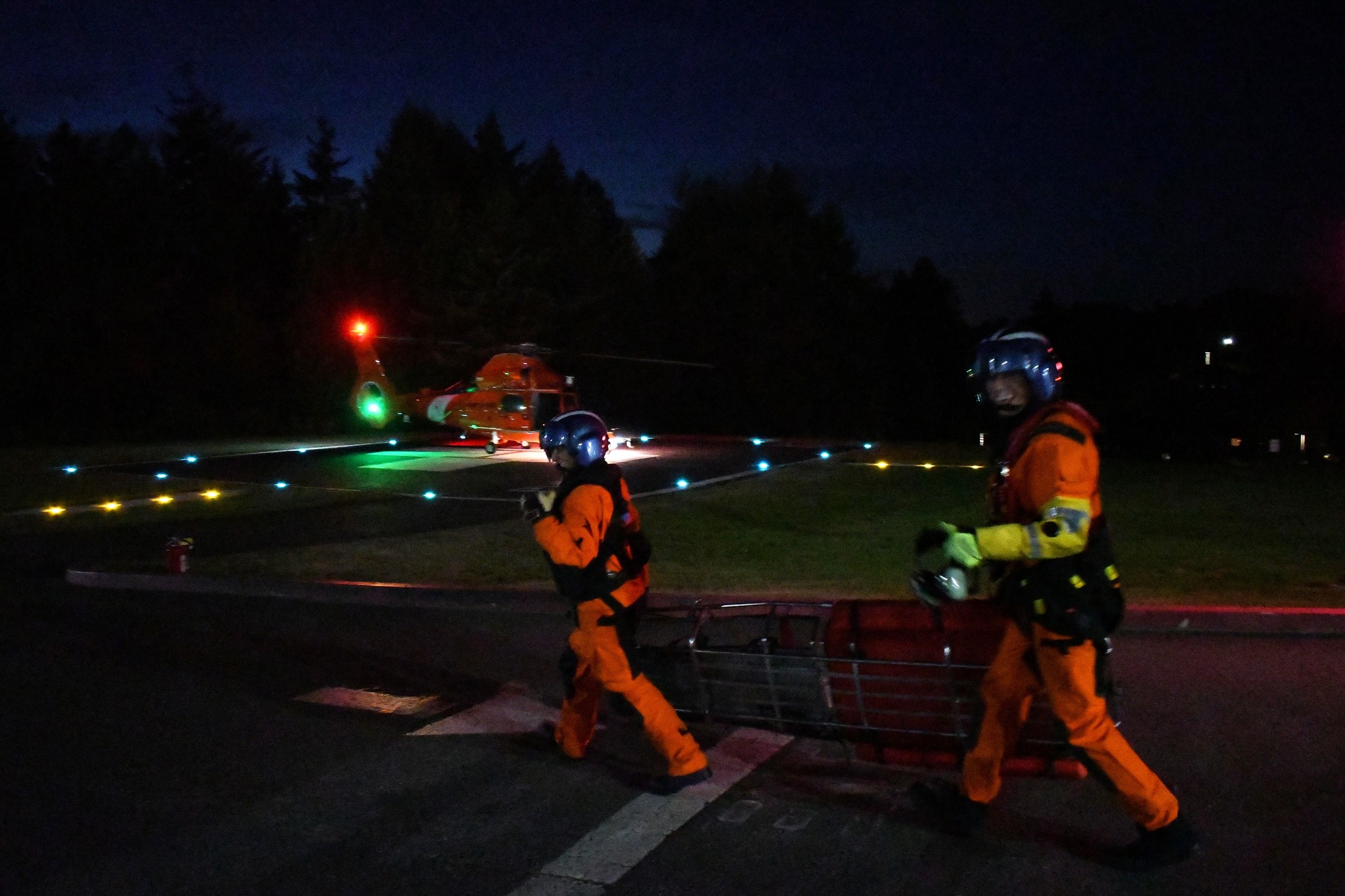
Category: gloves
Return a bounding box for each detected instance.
[939,521,984,570]
[517,491,556,521]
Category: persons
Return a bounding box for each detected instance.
[522,411,712,793]
[908,329,1197,871]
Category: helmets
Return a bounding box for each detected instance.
[973,327,1065,407]
[540,411,609,467]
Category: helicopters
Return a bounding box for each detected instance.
[350,320,716,454]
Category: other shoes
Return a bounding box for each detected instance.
[1107,819,1198,871]
[638,767,714,794]
[908,777,997,829]
[540,719,571,763]
